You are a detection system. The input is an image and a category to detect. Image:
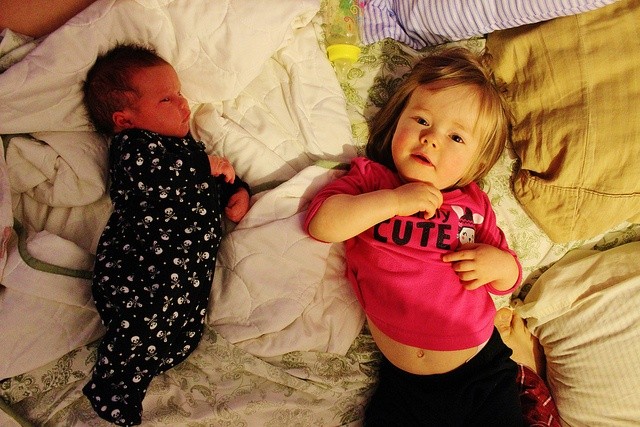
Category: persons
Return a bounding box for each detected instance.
[307,48,525,427]
[81,44,252,427]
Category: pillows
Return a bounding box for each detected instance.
[356,0,617,47]
[518,241,640,427]
[484,0,640,238]
